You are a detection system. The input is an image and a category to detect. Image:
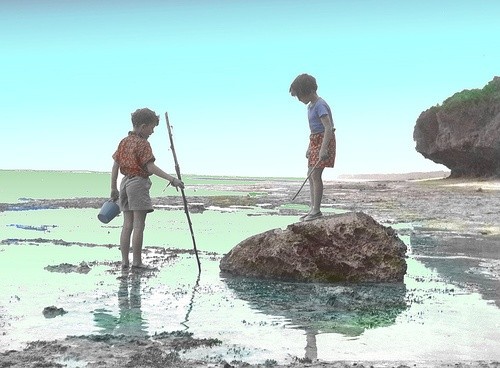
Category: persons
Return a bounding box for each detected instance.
[289,73,336,221]
[111,107,185,271]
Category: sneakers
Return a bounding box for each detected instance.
[300,211,323,221]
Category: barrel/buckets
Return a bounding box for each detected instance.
[98,201,120,223]
